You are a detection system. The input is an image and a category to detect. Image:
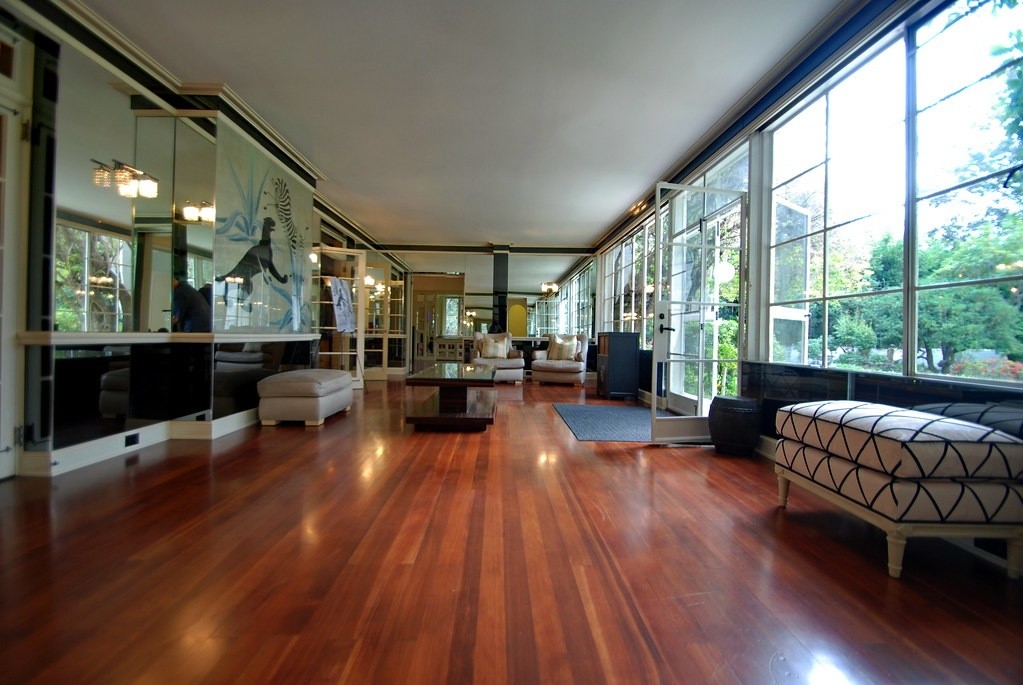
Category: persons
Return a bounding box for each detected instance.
[173,271,212,333]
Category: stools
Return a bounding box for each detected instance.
[257,368,353,426]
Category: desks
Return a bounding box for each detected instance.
[434,336,463,361]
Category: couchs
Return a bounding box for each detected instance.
[532,334,589,386]
[470,331,525,385]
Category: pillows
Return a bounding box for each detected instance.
[482,333,508,358]
[547,335,578,361]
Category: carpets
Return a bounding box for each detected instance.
[552,403,675,441]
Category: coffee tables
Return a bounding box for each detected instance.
[405,363,498,433]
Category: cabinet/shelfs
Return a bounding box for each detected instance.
[596,332,640,401]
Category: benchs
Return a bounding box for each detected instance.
[773,399,1022,579]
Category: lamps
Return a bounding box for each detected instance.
[87,157,112,187]
[112,159,160,198]
[182,199,213,221]
